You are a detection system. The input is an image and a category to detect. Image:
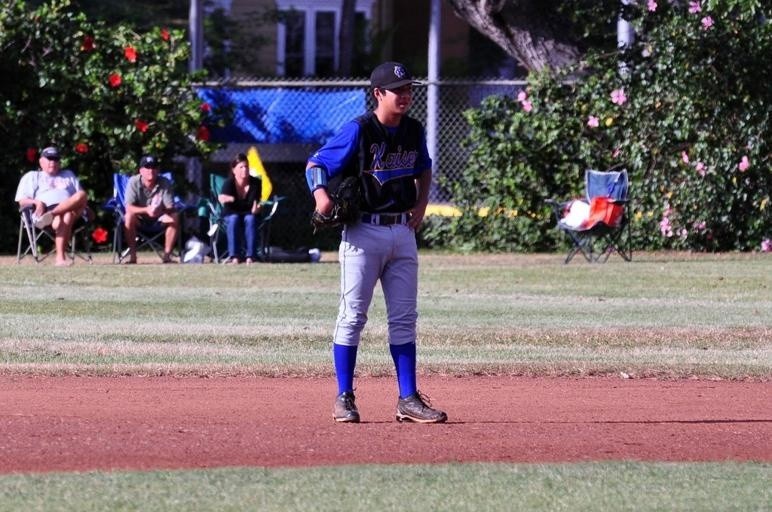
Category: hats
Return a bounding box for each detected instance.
[139,155,159,166]
[42,147,60,158]
[371,62,421,90]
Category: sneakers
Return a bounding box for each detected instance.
[333,392,360,422]
[36,212,53,229]
[395,391,448,423]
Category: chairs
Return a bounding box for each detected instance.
[197,172,282,264]
[106,172,189,263]
[14,168,92,265]
[545,168,633,263]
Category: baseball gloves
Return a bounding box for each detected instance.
[311,171,361,235]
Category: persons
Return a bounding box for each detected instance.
[217,151,262,267]
[303,59,450,428]
[12,145,97,267]
[119,153,180,264]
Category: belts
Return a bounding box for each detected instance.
[362,214,410,226]
